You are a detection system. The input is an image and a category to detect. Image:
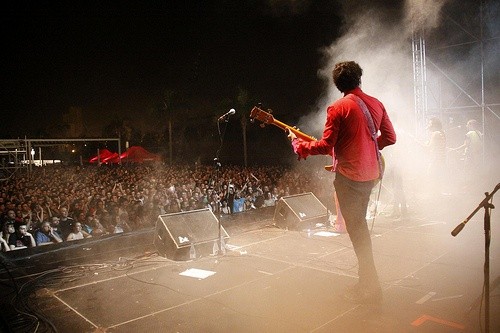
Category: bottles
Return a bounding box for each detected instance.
[221,236,226,256]
[189,243,196,261]
[213,240,219,256]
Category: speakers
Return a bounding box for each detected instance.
[153,208,230,262]
[272,192,331,232]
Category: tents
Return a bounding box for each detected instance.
[89,146,160,164]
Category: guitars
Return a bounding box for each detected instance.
[249,102,386,187]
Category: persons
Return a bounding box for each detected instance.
[285,61,397,302]
[67,222,92,241]
[405,117,448,158]
[37,220,63,245]
[0,230,10,252]
[0,159,337,237]
[9,219,36,250]
[55,207,75,238]
[449,119,485,169]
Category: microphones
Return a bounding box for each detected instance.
[219,109,236,120]
